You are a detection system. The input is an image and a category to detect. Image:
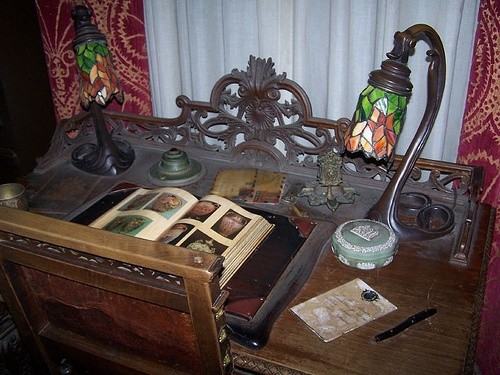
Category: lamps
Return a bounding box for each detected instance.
[60,11,138,175]
[344,22,462,243]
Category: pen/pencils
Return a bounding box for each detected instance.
[374,306,440,343]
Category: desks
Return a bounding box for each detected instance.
[6,57,492,373]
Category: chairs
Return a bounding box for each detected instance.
[0,205,235,373]
